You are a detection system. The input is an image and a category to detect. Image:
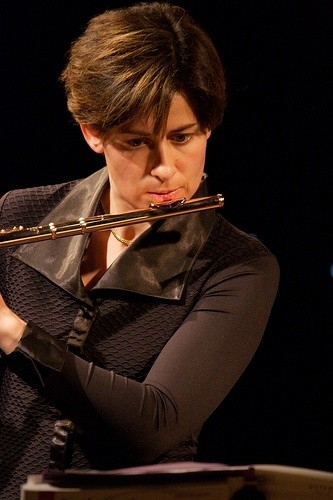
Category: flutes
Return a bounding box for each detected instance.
[0,193,225,248]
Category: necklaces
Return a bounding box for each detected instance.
[99,199,133,246]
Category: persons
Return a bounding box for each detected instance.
[0,2,278,500]
